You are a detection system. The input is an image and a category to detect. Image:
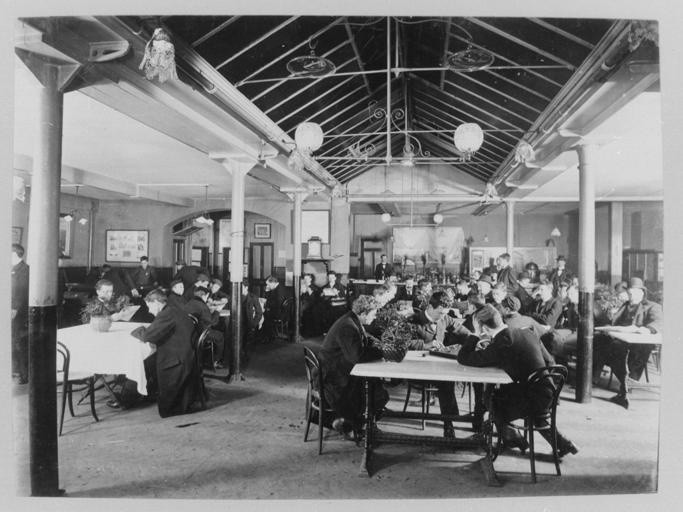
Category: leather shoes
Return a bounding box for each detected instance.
[340,416,497,443]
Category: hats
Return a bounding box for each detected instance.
[505,295,522,312]
[622,277,647,293]
[474,273,494,289]
[169,273,223,289]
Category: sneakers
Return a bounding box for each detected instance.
[106,399,121,410]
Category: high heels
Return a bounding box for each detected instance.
[556,441,579,457]
[505,437,530,451]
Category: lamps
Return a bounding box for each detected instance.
[444,37,495,73]
[294,121,324,158]
[286,35,337,80]
[381,213,391,223]
[549,225,561,237]
[453,121,485,161]
[433,213,443,224]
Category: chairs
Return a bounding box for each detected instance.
[55,295,296,437]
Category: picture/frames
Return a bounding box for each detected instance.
[11,226,23,246]
[289,208,331,245]
[105,229,150,262]
[254,222,272,239]
[57,212,76,259]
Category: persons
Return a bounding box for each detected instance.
[7,242,30,387]
[82,256,288,417]
[299,254,658,460]
